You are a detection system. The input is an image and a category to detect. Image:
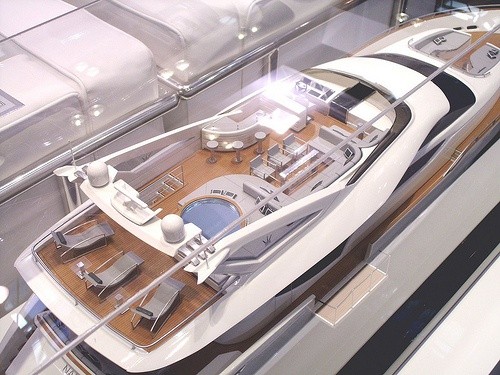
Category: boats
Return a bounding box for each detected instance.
[0,3,500,375]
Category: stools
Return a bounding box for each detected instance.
[207,141,218,163]
[232,141,243,162]
[255,132,267,153]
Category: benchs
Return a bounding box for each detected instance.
[242,126,362,210]
[330,124,384,146]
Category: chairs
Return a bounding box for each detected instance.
[85,250,145,303]
[268,143,295,171]
[283,133,308,160]
[249,153,277,182]
[50,218,115,264]
[130,277,185,338]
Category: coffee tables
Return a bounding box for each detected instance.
[68,255,93,279]
[105,286,132,314]
